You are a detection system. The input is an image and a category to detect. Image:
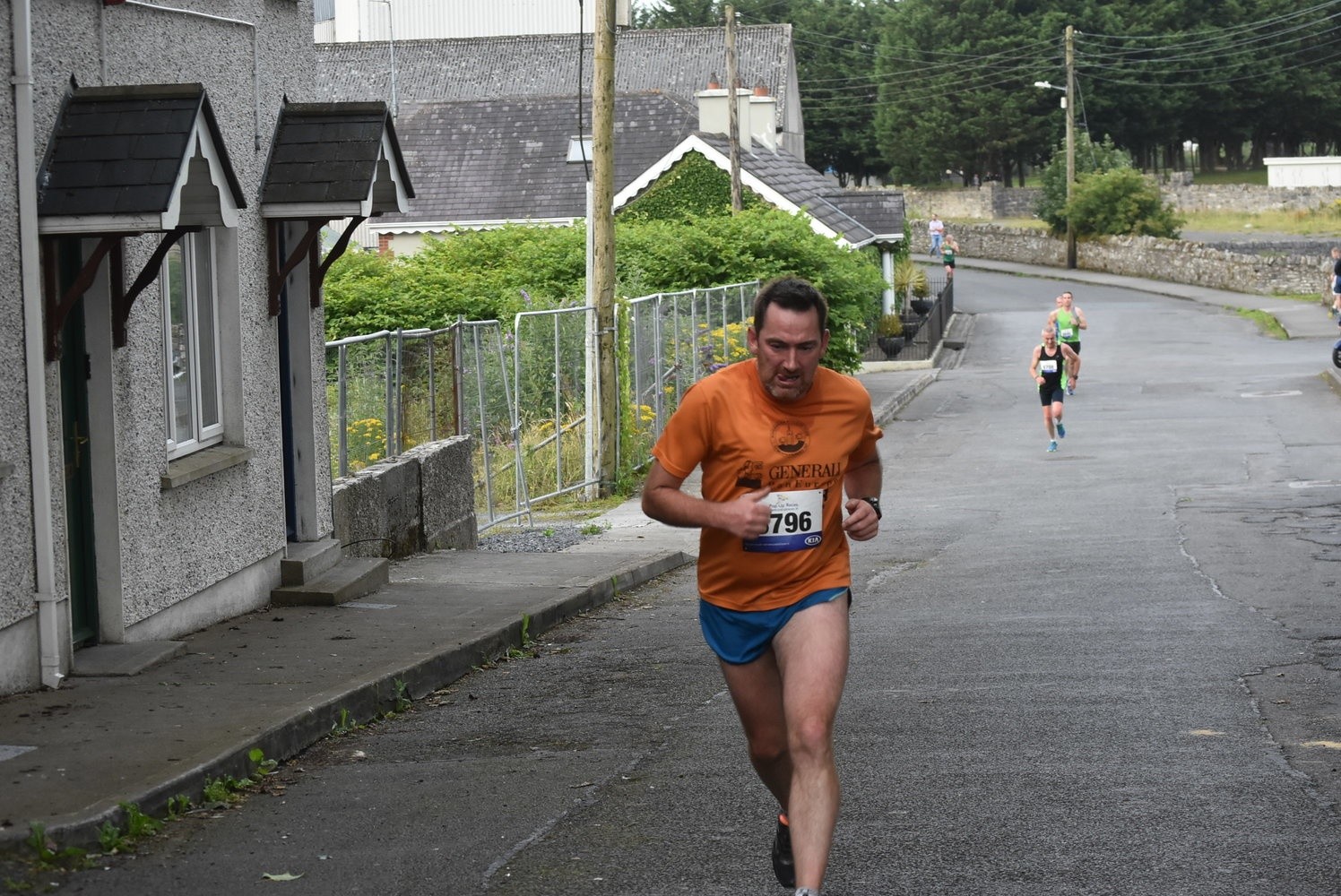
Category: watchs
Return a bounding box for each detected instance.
[860,496,882,520]
[1071,375,1078,380]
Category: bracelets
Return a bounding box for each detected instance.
[1034,376,1039,381]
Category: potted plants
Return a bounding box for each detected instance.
[876,312,905,360]
[911,264,933,316]
[890,256,922,346]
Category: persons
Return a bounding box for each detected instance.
[1029,326,1081,453]
[928,213,944,259]
[1048,292,1087,395]
[940,235,959,284]
[641,274,885,896]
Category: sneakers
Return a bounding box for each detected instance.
[772,813,794,887]
[1047,441,1057,452]
[1057,423,1065,438]
[1067,384,1073,395]
